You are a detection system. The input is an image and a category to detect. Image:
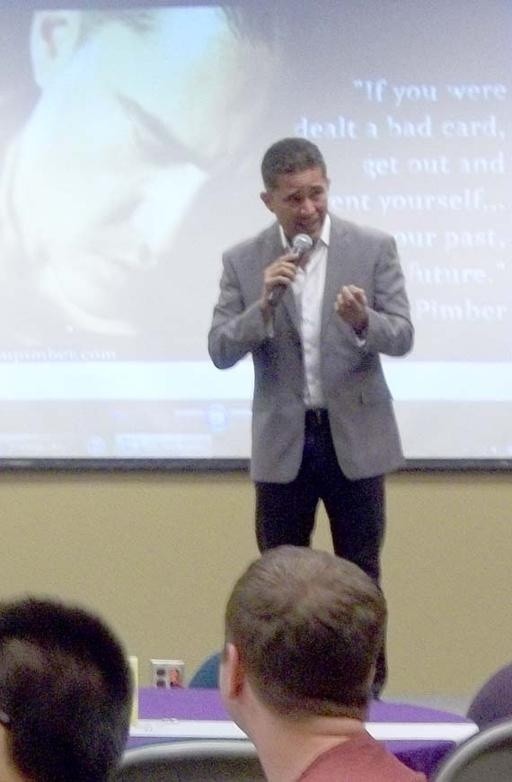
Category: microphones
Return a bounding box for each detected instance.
[265,232,313,308]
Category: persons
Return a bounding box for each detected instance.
[206,136,414,703]
[0,589,135,780]
[1,6,331,347]
[216,543,434,781]
